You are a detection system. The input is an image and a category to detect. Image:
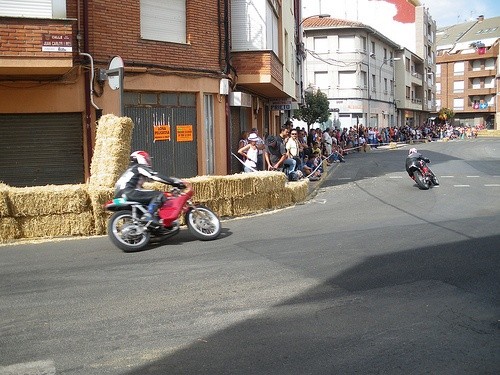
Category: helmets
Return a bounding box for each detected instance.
[409,148,417,154]
[130,151,152,168]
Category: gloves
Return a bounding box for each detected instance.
[172,181,186,189]
[425,158,430,163]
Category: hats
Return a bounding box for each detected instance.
[266,135,277,146]
[248,133,258,140]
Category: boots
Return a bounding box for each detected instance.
[141,202,164,226]
[422,167,432,177]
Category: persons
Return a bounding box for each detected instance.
[405,148,429,176]
[239,119,494,182]
[238,133,265,173]
[114,150,187,224]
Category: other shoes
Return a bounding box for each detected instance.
[340,159,346,162]
[309,176,321,181]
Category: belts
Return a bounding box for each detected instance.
[326,142,331,145]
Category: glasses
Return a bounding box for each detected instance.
[291,133,297,135]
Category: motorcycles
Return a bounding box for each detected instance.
[103,182,222,253]
[407,156,439,190]
